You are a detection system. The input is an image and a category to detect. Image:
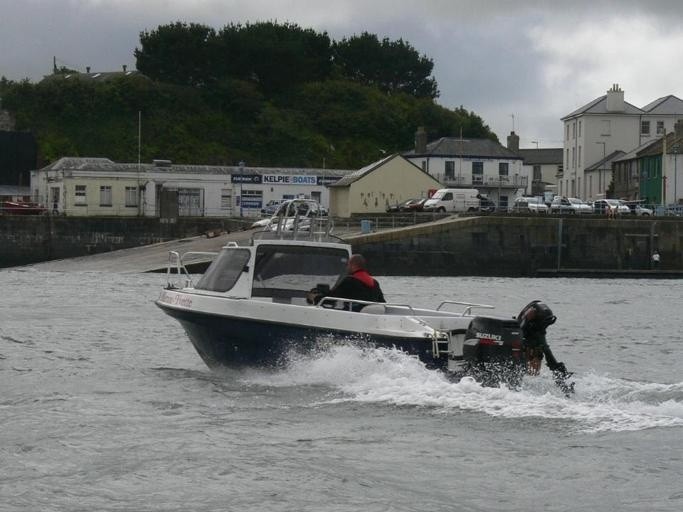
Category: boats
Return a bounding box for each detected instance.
[153,197,581,400]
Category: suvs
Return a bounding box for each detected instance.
[256,195,333,233]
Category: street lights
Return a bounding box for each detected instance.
[237,158,246,218]
[531,140,540,186]
[594,141,608,200]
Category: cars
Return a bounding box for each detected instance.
[1,201,47,215]
[386,186,680,217]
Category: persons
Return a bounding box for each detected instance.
[305,253,387,313]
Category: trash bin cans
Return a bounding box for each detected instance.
[361,220,371,234]
[595,202,606,215]
